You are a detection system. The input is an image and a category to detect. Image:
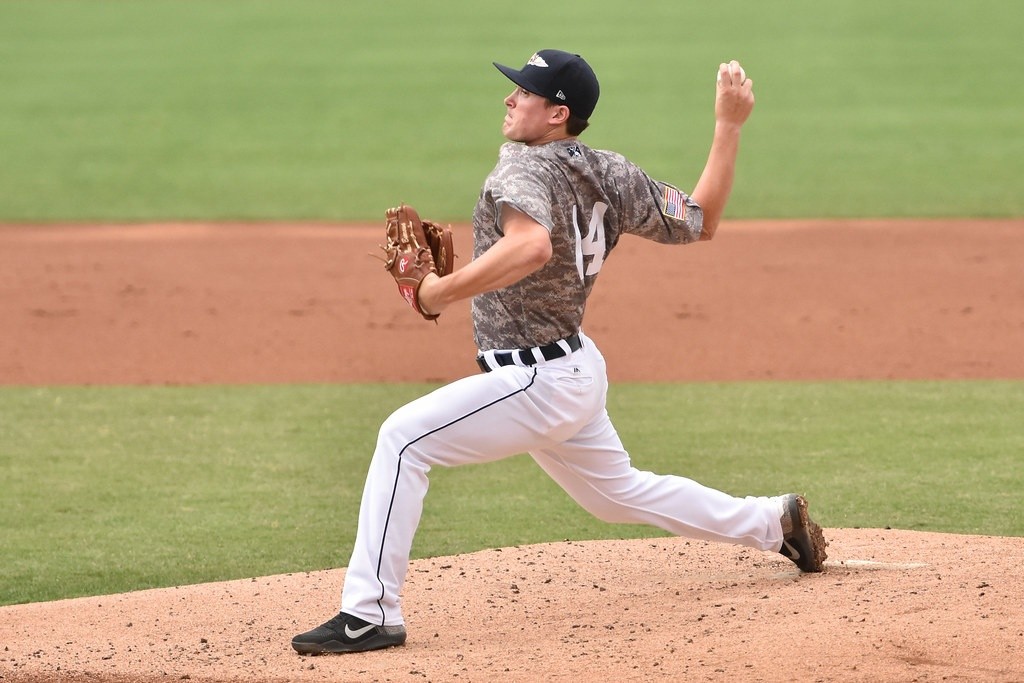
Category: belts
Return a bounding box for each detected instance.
[475,334,581,372]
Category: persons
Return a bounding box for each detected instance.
[289,50,828,659]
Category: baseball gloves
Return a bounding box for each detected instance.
[365,199,461,327]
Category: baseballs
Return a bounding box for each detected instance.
[716,63,746,86]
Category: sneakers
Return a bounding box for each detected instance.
[292,611,407,656]
[778,493,828,572]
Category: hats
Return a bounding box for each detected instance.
[493,49,600,120]
